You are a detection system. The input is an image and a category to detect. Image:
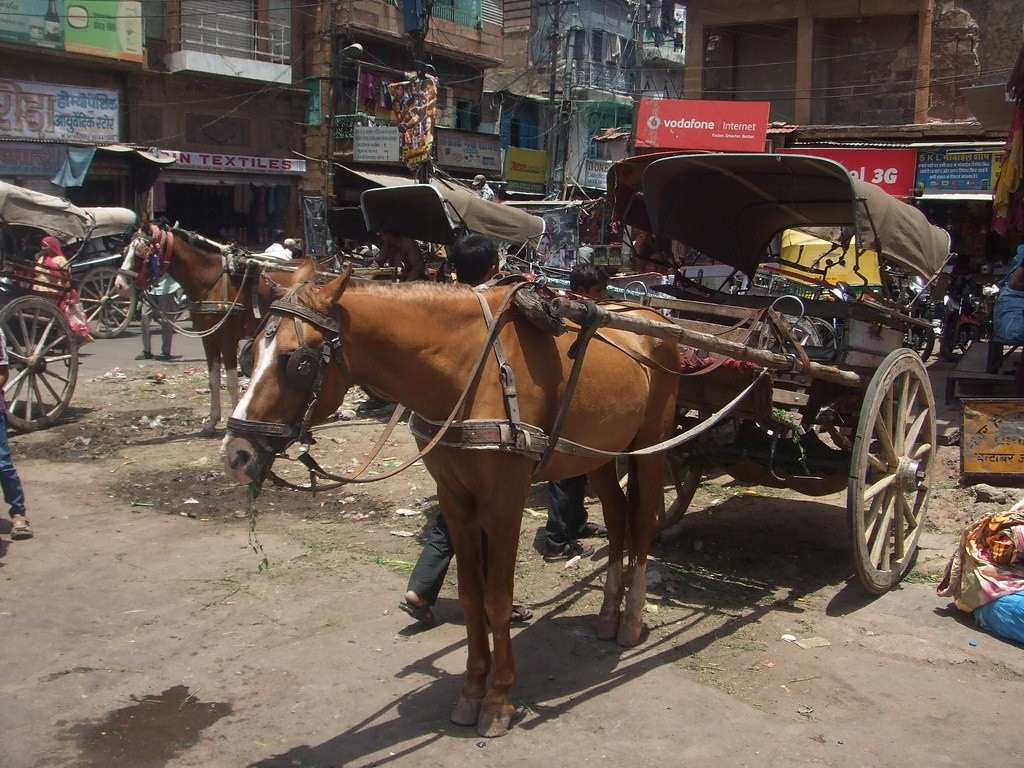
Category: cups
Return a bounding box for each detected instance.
[29,24,45,43]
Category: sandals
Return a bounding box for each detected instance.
[11,514,33,539]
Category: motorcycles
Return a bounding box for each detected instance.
[881,261,1001,363]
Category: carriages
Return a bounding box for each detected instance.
[115,158,546,437]
[220,152,953,738]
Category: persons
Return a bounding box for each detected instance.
[136,217,182,360]
[374,217,425,281]
[578,240,594,264]
[0,328,33,539]
[544,264,610,563]
[993,244,1024,341]
[266,229,303,260]
[472,175,494,201]
[394,79,437,149]
[35,237,71,306]
[399,234,533,625]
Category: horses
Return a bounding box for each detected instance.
[218,253,682,740]
[114,210,375,439]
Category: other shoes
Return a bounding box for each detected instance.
[357,398,383,410]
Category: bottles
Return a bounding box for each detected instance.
[237,226,268,245]
[44,0,60,42]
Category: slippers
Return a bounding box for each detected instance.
[397,597,435,626]
[545,540,594,562]
[511,603,533,621]
[156,350,170,361]
[136,352,152,359]
[578,523,609,539]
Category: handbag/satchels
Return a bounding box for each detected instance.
[59,302,90,338]
